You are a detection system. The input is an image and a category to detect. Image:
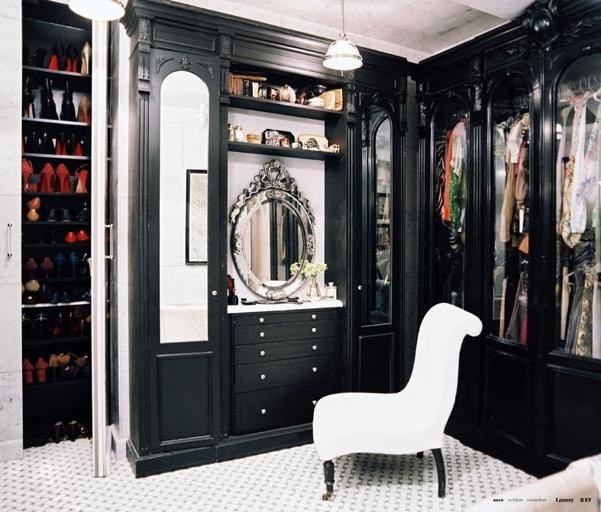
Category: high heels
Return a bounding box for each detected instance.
[21,40,90,449]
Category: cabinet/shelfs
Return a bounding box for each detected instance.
[411,0,601,480]
[20,0,112,452]
[125,0,412,477]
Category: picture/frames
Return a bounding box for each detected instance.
[185,168,208,266]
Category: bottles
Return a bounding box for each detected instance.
[234,124,243,142]
[228,124,234,141]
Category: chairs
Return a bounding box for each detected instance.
[310,301,485,502]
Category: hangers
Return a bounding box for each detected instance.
[493,94,530,146]
[555,74,601,126]
[436,109,472,140]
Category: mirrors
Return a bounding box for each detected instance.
[155,58,212,349]
[360,90,397,330]
[228,158,319,306]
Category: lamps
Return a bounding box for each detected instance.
[67,0,127,22]
[322,0,364,72]
